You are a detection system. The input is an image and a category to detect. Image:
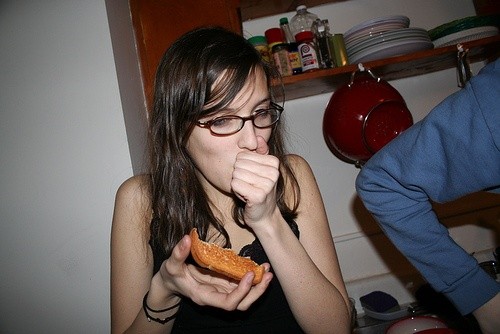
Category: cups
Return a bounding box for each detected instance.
[328,33,349,67]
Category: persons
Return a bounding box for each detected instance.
[110,25,354,334]
[355,57,500,334]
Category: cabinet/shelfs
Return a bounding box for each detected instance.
[105,0,500,128]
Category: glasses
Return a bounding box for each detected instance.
[195,101,284,137]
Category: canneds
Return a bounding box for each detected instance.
[271,31,321,79]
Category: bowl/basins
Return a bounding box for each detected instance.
[323,68,413,168]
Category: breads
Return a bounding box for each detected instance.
[190,228,265,284]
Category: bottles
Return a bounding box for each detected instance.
[290,5,321,74]
[312,18,336,69]
[280,17,303,75]
[247,28,293,77]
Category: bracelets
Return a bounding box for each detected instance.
[143,291,183,324]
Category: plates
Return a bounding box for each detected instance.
[428,14,499,49]
[343,15,433,65]
